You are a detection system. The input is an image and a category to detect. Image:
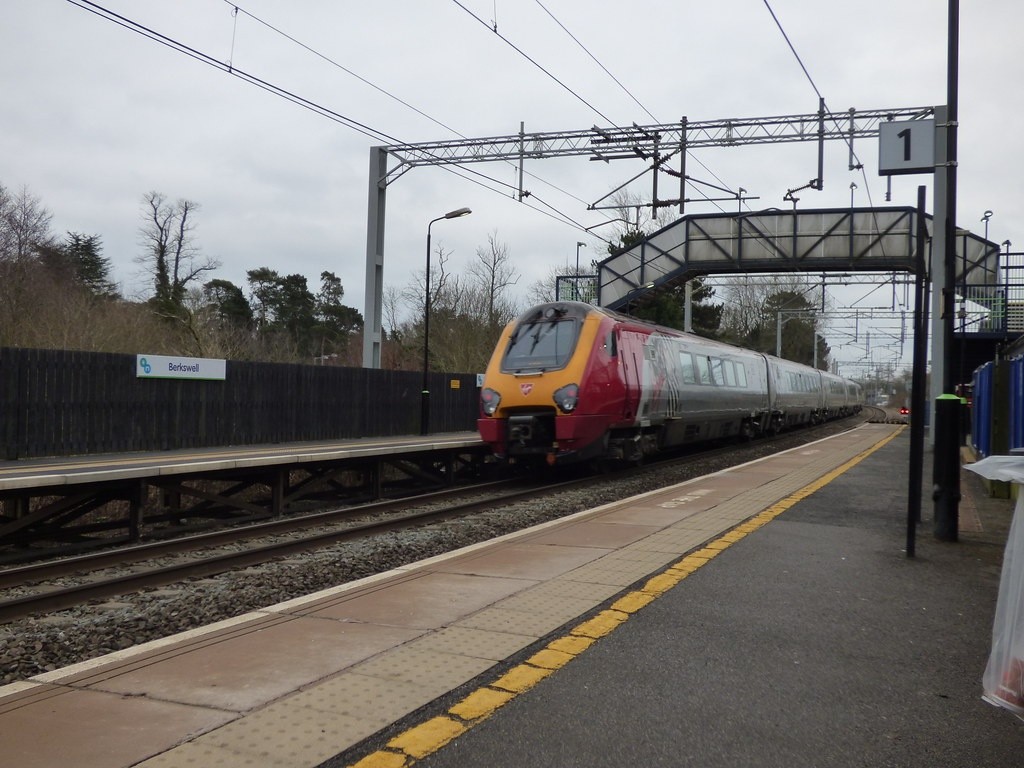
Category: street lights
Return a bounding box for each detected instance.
[418,207,471,437]
[1001,240,1012,370]
[575,242,586,301]
[981,211,991,306]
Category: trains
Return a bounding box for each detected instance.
[476,300,864,475]
